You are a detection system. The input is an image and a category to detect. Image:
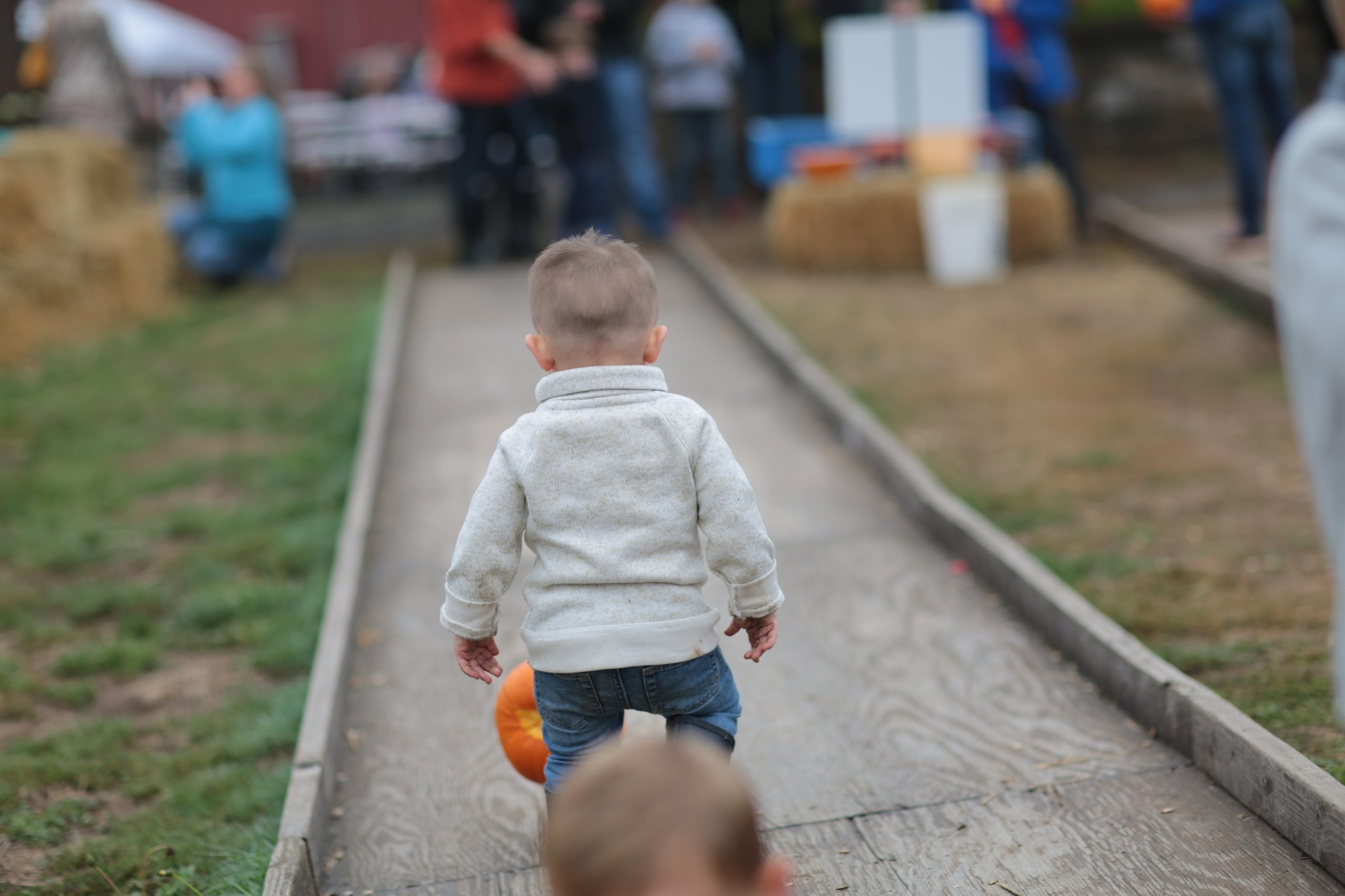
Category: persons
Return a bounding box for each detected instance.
[441,227,786,813]
[0,1,1096,275]
[537,726,789,896]
[1273,1,1345,721]
[1187,0,1301,238]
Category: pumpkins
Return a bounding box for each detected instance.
[494,660,625,783]
